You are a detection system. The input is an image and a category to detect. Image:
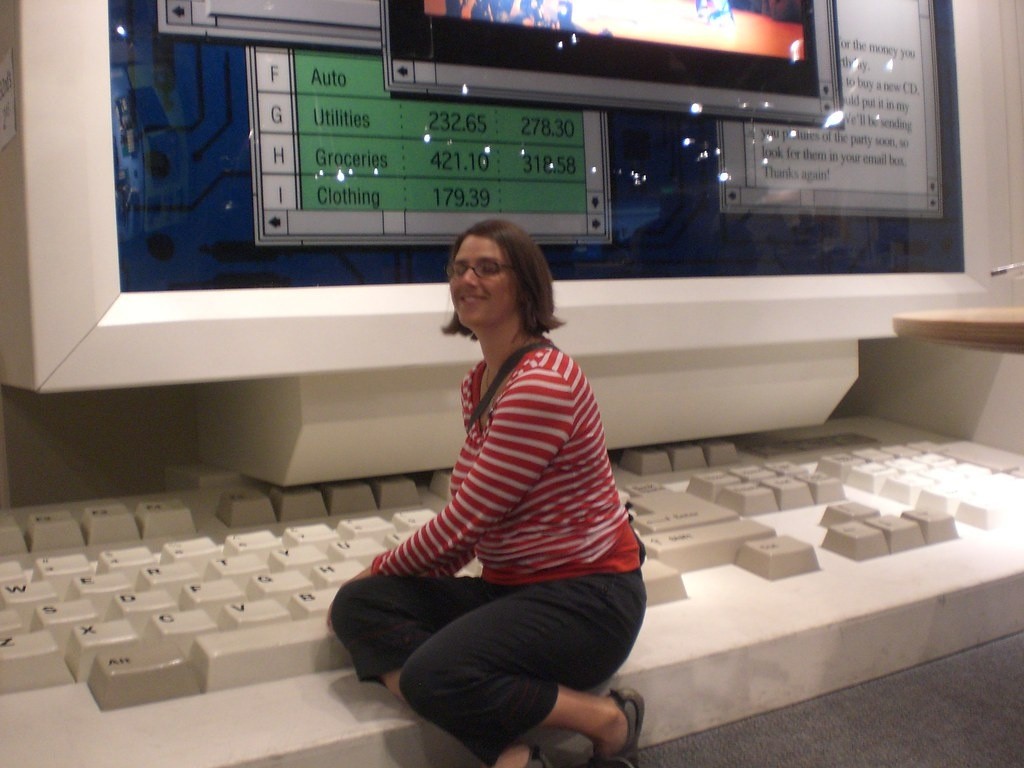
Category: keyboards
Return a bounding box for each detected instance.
[0,411,1024,768]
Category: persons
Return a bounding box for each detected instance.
[327,221,649,768]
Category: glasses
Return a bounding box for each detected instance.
[445,258,514,279]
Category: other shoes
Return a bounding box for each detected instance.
[589,687,644,768]
[521,742,554,768]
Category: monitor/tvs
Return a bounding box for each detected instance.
[0,0,1024,485]
